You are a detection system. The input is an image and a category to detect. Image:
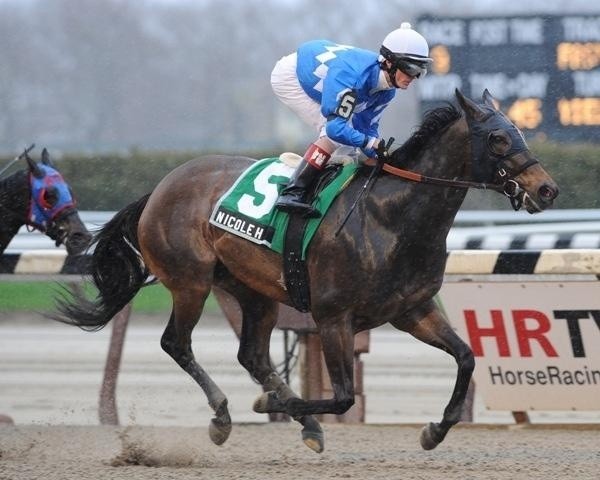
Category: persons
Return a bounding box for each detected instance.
[262,17,431,217]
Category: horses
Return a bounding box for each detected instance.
[0,144,93,260]
[33,88,559,453]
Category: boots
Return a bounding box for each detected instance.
[276,143,331,218]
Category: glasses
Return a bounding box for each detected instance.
[380,47,427,80]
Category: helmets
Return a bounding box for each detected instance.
[379,23,433,65]
